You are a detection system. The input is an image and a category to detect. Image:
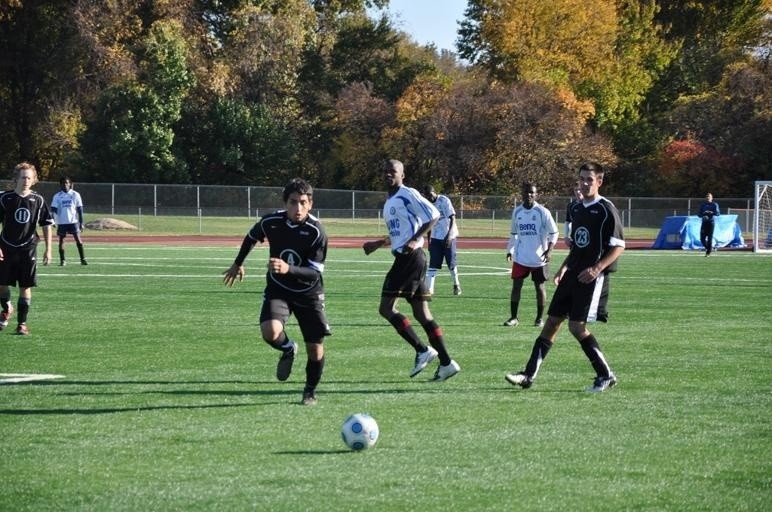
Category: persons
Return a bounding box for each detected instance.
[1,160,53,336]
[504,161,626,394]
[363,157,462,383]
[572,178,583,198]
[50,175,89,268]
[420,184,462,298]
[697,192,721,259]
[505,182,559,331]
[220,178,334,407]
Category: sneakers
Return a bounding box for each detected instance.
[0,310,13,330]
[302,386,318,404]
[705,252,711,257]
[505,318,520,326]
[454,282,462,296]
[60,259,65,266]
[409,345,439,378]
[430,360,461,382]
[81,259,89,265]
[505,372,533,390]
[590,372,617,393]
[17,323,28,335]
[276,342,298,381]
[534,319,545,327]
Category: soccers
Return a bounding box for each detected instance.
[340,413,379,450]
